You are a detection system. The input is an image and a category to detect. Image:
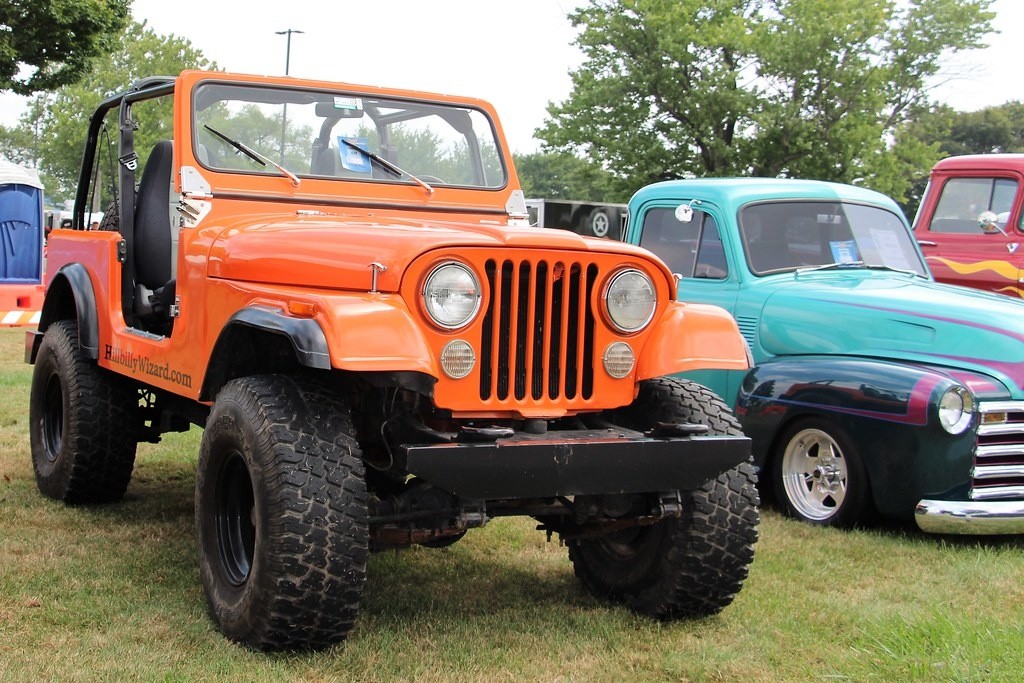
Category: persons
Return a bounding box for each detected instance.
[44,226,52,257]
[92,222,98,230]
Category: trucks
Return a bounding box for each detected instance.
[675,152,1024,301]
[523,174,1024,539]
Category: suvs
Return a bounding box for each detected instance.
[24,67,756,650]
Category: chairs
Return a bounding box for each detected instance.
[315,148,388,182]
[134,140,214,321]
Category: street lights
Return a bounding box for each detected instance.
[274,29,305,168]
